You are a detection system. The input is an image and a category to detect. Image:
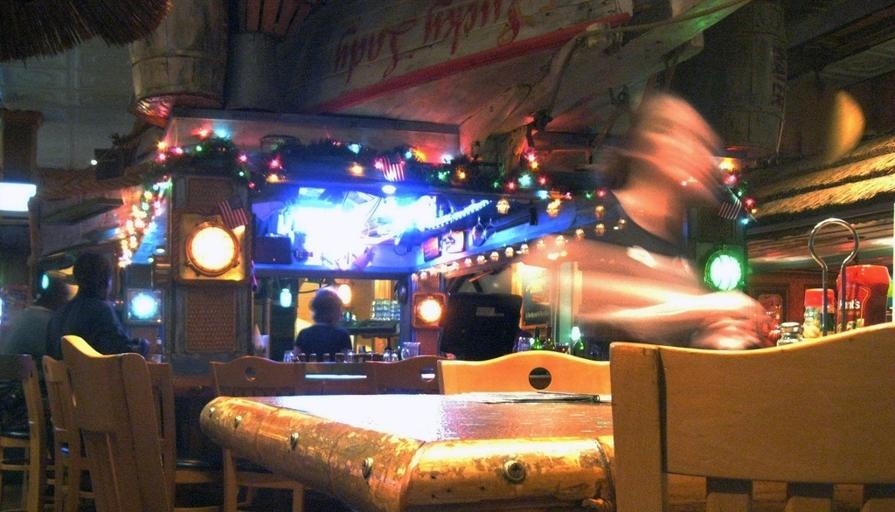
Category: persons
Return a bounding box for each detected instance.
[45,253,155,362]
[489,89,772,353]
[293,287,353,363]
[1,277,71,432]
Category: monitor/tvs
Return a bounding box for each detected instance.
[438,292,523,360]
[122,288,165,326]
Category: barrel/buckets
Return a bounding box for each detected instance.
[225,29,282,112]
[126,0,231,119]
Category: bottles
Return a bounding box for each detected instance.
[369,299,400,322]
[383,348,400,363]
[776,320,802,348]
[802,286,835,343]
[530,326,544,351]
[834,263,891,337]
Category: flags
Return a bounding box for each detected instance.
[380,151,404,183]
[217,194,248,230]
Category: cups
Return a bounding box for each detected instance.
[400,341,422,360]
